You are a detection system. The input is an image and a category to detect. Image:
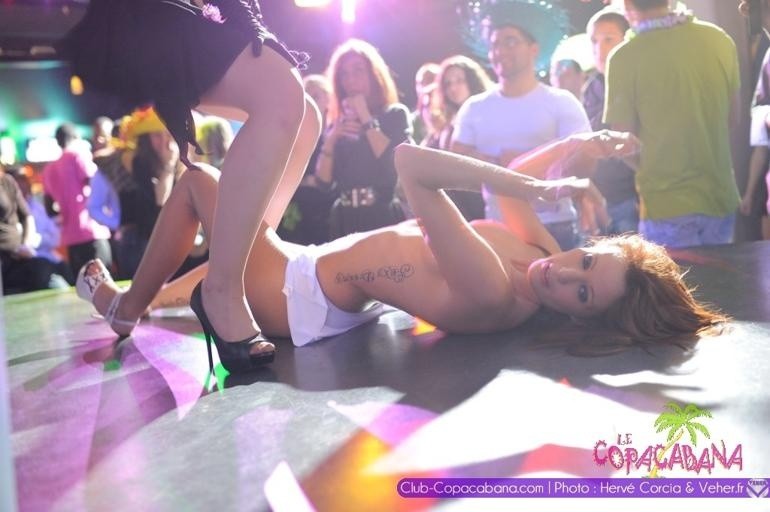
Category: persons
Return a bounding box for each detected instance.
[0,0,770,374]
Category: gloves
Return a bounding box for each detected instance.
[205,0,310,73]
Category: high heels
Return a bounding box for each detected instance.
[77,257,152,338]
[189,278,276,375]
